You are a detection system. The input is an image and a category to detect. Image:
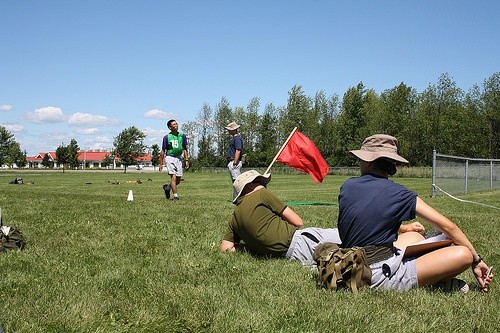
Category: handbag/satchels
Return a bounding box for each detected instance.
[312,242,371,295]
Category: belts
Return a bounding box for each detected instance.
[227,158,231,161]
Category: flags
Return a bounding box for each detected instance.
[276,129,331,184]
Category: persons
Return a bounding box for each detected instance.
[220,170,342,271]
[225,122,243,202]
[337,134,494,293]
[159,118,189,201]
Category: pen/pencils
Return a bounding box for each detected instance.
[481,266,494,293]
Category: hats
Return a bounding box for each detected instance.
[350,134,410,164]
[232,171,271,204]
[225,122,240,131]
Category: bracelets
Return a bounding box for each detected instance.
[185,159,189,161]
[233,163,238,165]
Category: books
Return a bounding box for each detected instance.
[400,232,454,262]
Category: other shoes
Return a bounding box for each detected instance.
[163,184,170,199]
[173,198,179,202]
[448,277,469,293]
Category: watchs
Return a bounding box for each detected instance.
[472,253,484,266]
